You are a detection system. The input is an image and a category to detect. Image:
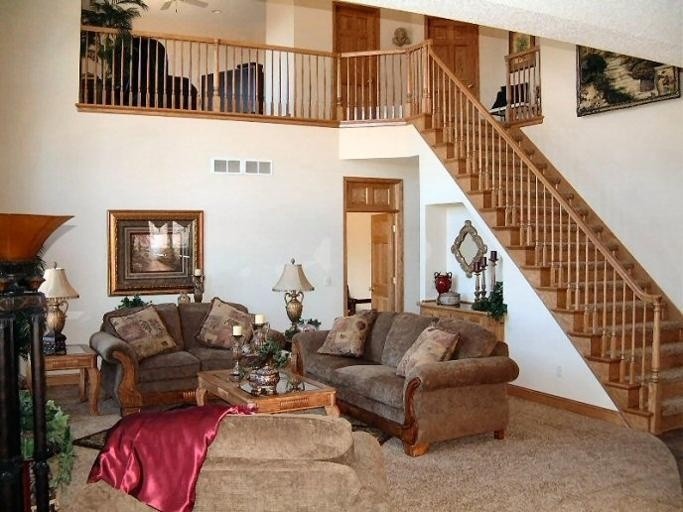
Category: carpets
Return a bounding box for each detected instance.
[16,373,683,511]
[72,405,394,451]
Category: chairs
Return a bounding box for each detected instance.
[87,406,387,511]
[104,38,196,110]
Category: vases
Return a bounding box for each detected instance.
[176,290,189,306]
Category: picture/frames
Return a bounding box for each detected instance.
[575,46,680,116]
[106,210,203,295]
[508,32,535,74]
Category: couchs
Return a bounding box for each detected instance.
[90,301,285,417]
[292,307,518,455]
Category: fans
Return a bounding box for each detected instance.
[159,0,207,11]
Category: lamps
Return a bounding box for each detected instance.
[0,211,75,511]
[271,258,315,338]
[38,262,80,354]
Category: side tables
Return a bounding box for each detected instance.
[283,335,292,353]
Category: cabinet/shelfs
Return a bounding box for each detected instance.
[417,299,505,342]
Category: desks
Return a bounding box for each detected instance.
[201,69,263,116]
[26,344,99,415]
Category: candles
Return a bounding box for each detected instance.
[255,314,264,323]
[195,268,201,275]
[232,326,241,335]
[473,250,497,271]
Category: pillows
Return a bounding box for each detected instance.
[317,309,378,357]
[106,304,177,362]
[195,297,253,350]
[395,322,459,376]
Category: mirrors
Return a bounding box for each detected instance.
[450,220,487,278]
